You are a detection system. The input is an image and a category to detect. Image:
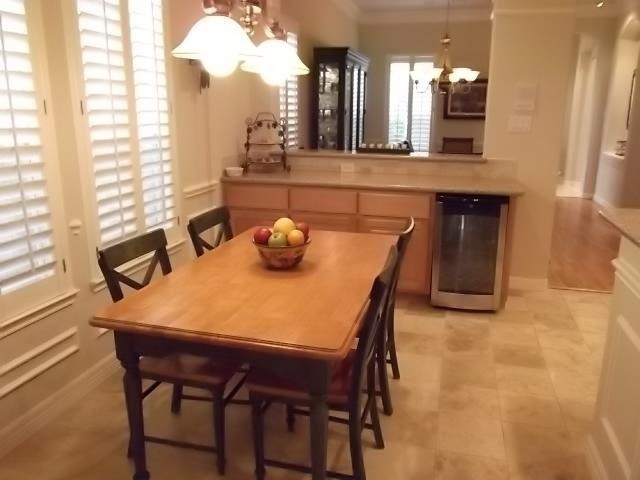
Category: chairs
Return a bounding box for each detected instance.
[97,229,243,475]
[244,245,399,479]
[186,206,233,259]
[353,215,416,416]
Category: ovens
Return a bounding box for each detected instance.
[429,195,511,312]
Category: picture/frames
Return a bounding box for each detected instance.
[443,79,488,118]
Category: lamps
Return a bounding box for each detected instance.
[170,0,311,88]
[406,0,481,97]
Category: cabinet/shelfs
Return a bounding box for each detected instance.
[579,235,640,480]
[288,186,358,233]
[221,182,288,240]
[313,47,371,151]
[358,188,434,297]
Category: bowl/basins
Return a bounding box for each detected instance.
[225,166,244,177]
[252,238,312,271]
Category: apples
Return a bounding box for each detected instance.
[268,233,287,247]
[254,227,271,245]
[296,222,309,235]
[287,230,304,245]
[273,216,295,235]
